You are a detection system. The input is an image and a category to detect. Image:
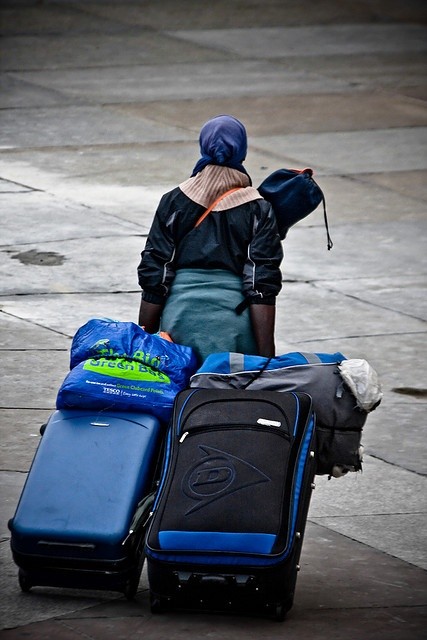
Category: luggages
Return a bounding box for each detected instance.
[5,407,165,604]
[143,389,317,618]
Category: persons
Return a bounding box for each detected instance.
[137,113,284,358]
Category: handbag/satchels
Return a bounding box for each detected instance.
[191,351,382,478]
[56,317,193,420]
